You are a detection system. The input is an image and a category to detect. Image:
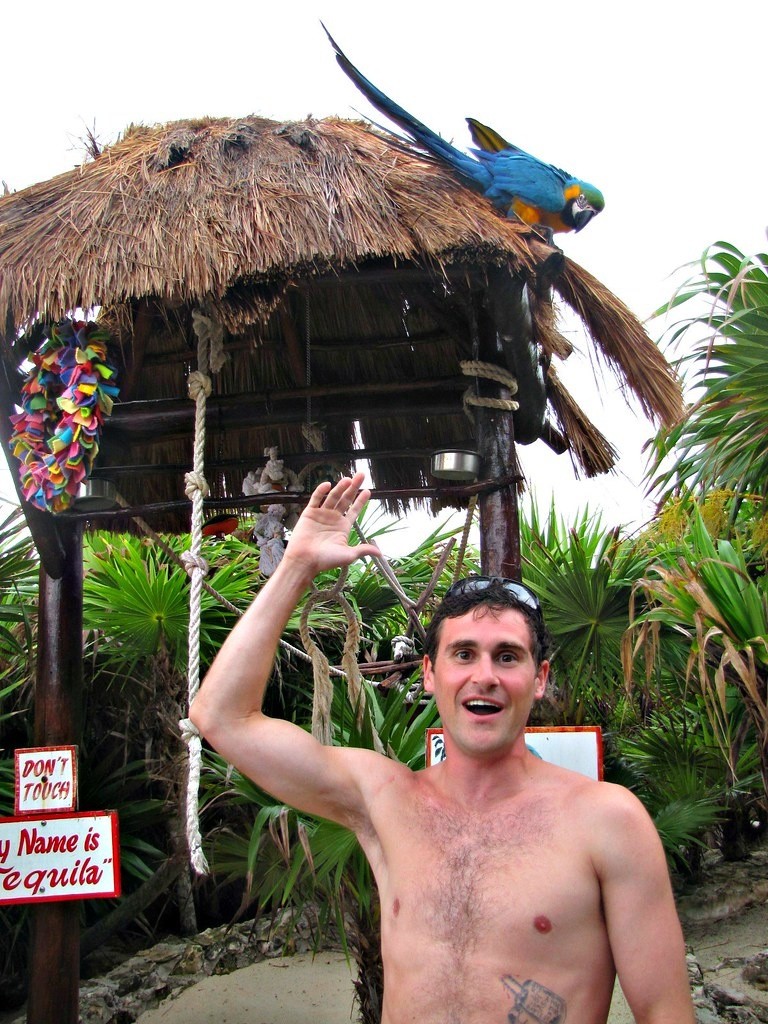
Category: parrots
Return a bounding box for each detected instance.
[321,20,605,256]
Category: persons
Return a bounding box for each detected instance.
[187,471,698,1024]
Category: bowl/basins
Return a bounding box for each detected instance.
[430,448,483,481]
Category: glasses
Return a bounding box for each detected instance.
[444,576,543,620]
